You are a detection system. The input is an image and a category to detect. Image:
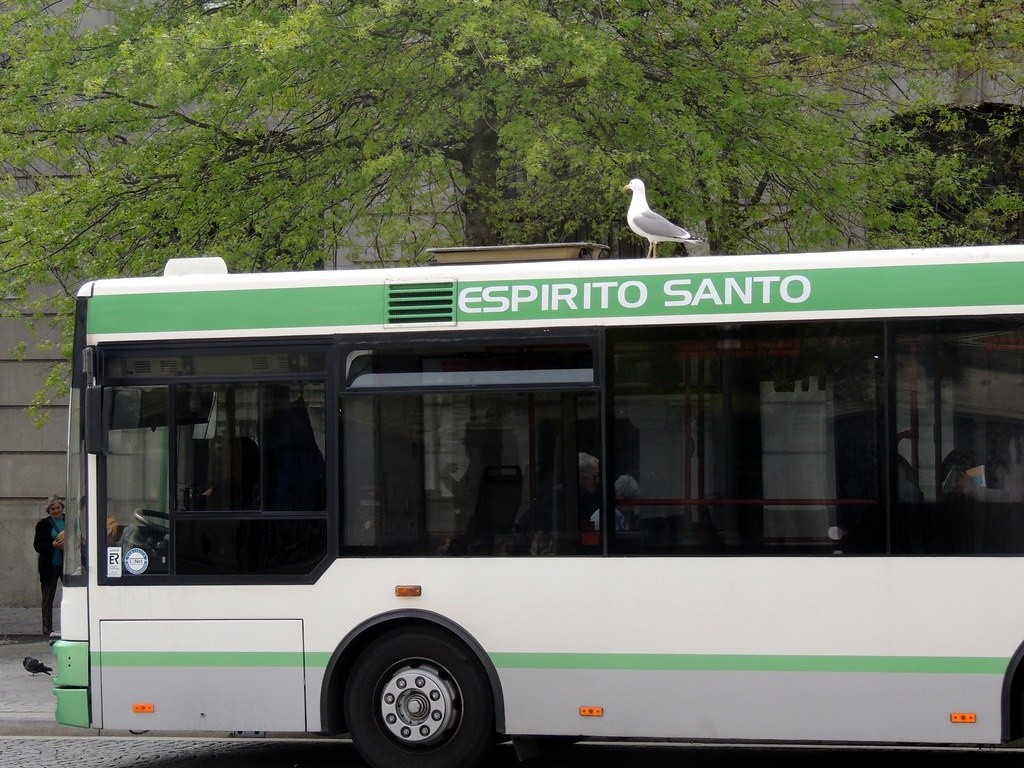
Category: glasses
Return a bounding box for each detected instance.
[582,472,600,481]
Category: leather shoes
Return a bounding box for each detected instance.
[42,626,55,633]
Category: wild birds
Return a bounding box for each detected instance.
[623,178,708,259]
[23,657,53,676]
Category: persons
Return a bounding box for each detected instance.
[31,494,67,638]
[543,452,601,529]
[201,437,284,510]
[589,475,646,533]
[439,420,495,558]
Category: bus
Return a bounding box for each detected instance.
[55,239,1023,768]
